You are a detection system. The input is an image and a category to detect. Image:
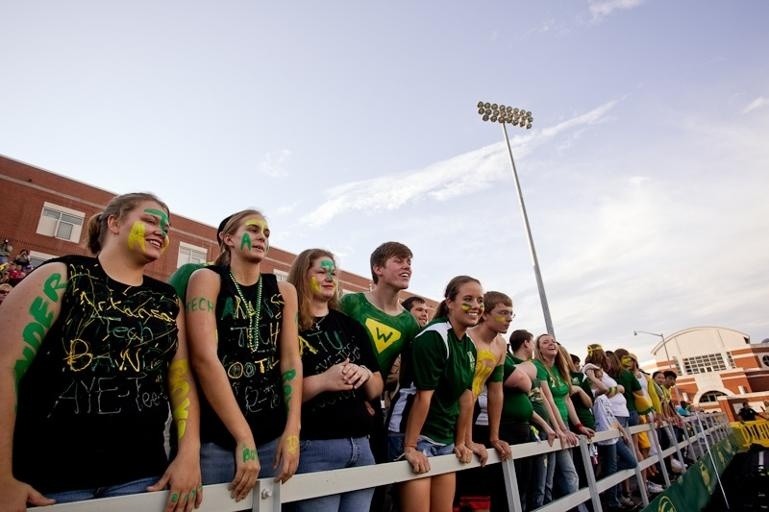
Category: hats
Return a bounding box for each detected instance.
[628,353,638,365]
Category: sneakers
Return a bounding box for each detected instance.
[609,456,694,512]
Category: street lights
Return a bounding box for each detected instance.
[633,329,672,369]
[477,99,555,352]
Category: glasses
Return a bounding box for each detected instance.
[489,311,516,316]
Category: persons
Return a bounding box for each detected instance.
[1,193,203,512]
[736,400,769,440]
[405,276,686,512]
[1,239,33,302]
[288,249,385,512]
[337,241,420,512]
[673,399,726,441]
[168,209,303,512]
